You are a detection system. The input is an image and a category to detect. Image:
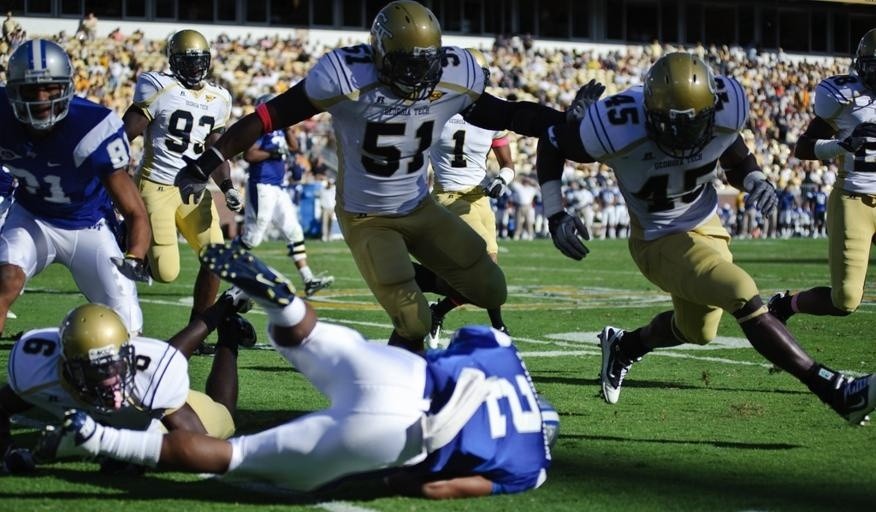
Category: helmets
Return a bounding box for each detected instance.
[855,29,876,89]
[371,0,442,100]
[59,303,137,412]
[256,94,275,106]
[539,399,560,446]
[169,30,210,83]
[643,53,719,159]
[467,48,490,91]
[6,39,75,130]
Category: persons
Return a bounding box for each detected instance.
[427,48,514,348]
[7,304,237,498]
[26,243,560,501]
[471,33,848,240]
[0,10,336,241]
[0,38,154,338]
[767,28,876,325]
[174,0,605,353]
[536,52,876,427]
[234,126,336,296]
[119,29,243,356]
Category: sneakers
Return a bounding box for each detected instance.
[198,244,297,306]
[597,326,642,404]
[834,374,876,427]
[192,286,256,356]
[115,221,126,253]
[31,408,105,464]
[305,270,334,297]
[426,298,445,349]
[768,290,795,326]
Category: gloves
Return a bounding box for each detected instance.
[4,448,34,474]
[548,211,589,260]
[110,255,154,287]
[220,179,243,213]
[837,136,867,152]
[567,79,605,119]
[743,170,779,218]
[174,163,208,204]
[485,177,506,198]
